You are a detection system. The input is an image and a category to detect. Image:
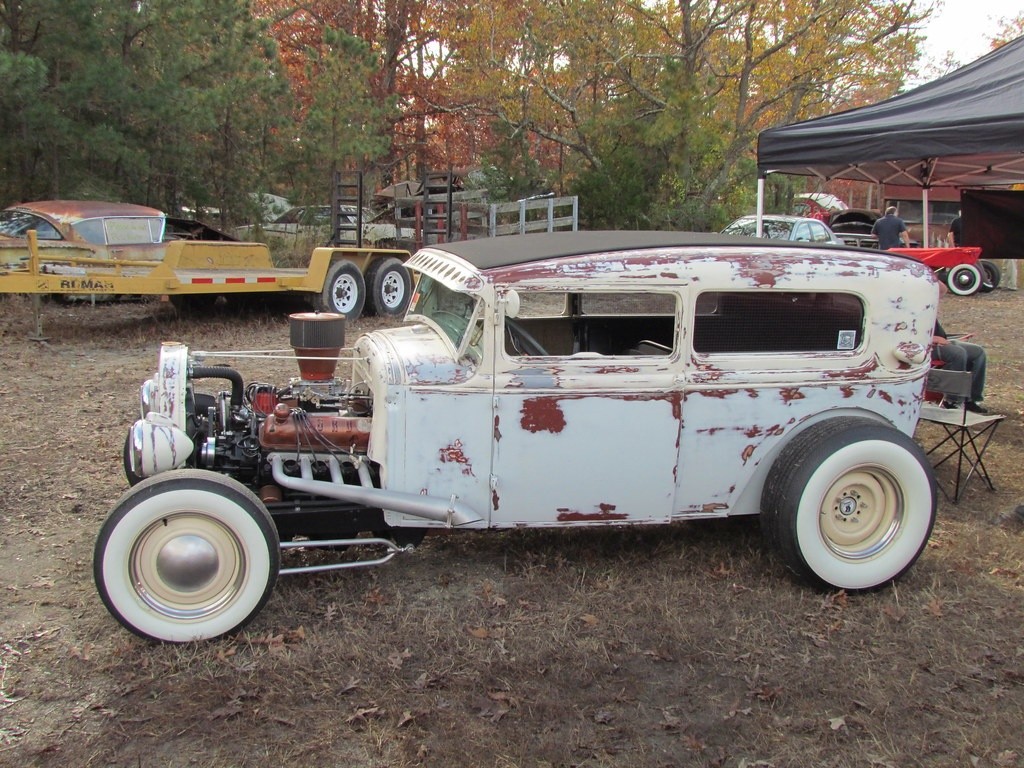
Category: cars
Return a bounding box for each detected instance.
[91,232,940,647]
[711,214,845,247]
[0,200,239,307]
[180,189,424,251]
[794,193,849,226]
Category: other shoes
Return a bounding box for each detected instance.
[939,398,958,409]
[964,401,987,413]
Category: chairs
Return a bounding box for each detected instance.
[924,332,973,407]
[914,372,1006,503]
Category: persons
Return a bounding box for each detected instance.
[931,280,988,413]
[871,206,909,251]
[948,217,960,247]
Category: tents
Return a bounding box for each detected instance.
[755,34,1024,249]
